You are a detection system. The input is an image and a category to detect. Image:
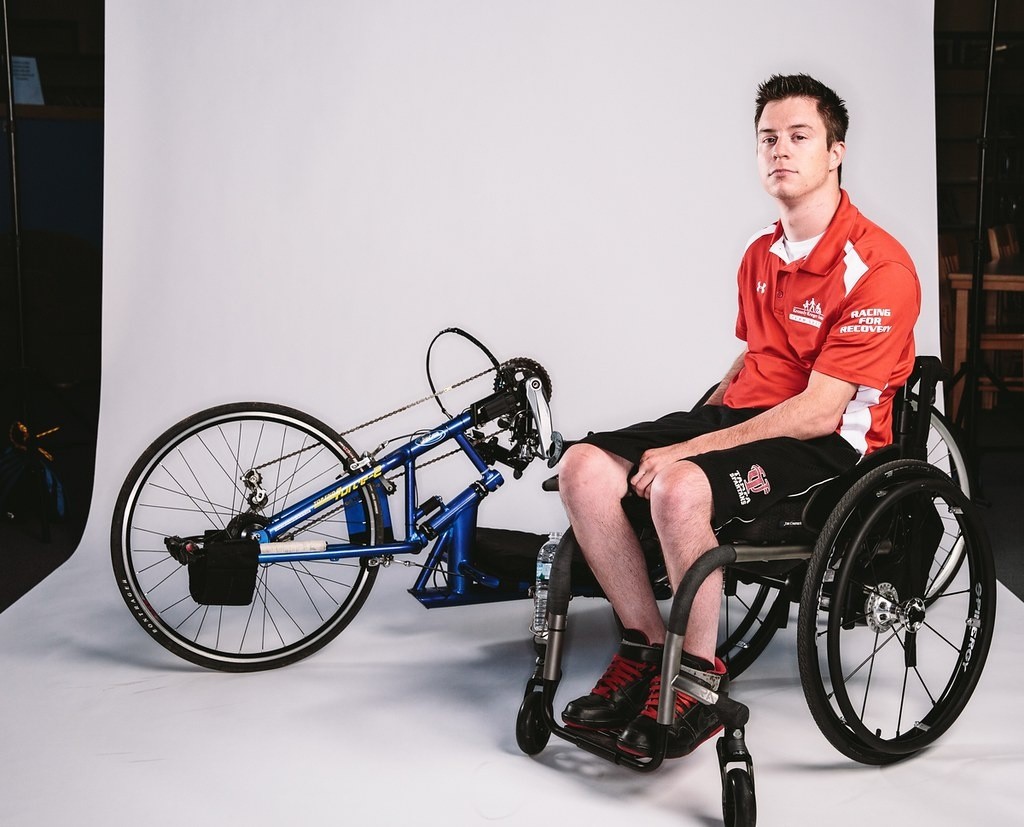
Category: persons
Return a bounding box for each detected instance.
[555,71,921,760]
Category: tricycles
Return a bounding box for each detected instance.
[109,331,982,674]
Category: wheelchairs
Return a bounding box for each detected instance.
[514,349,998,827]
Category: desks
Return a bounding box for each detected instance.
[950,260,1024,429]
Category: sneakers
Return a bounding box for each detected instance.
[617,650,731,759]
[561,628,666,731]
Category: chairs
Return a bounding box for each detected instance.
[939,222,1024,429]
